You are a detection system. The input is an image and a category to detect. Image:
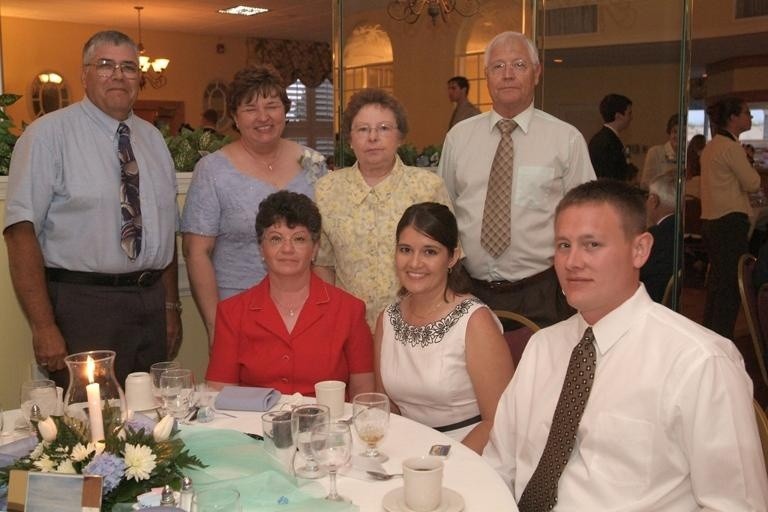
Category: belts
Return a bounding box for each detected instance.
[46,268,165,288]
[459,264,556,295]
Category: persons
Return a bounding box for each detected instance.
[313,87,467,342]
[182,65,336,349]
[6,30,183,395]
[435,30,599,332]
[376,202,513,456]
[206,188,375,407]
[447,75,480,127]
[482,178,768,510]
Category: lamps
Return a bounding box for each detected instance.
[386,0,480,30]
[131,6,169,90]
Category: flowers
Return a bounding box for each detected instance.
[0,400,207,512]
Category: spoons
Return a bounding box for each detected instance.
[365,468,404,481]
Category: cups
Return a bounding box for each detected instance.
[189,488,241,512]
[401,456,443,510]
[314,380,347,416]
[0,380,64,437]
[126,359,195,421]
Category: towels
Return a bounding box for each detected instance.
[216,385,279,411]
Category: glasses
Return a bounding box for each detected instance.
[486,59,535,74]
[83,60,144,80]
[349,122,399,139]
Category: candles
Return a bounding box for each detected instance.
[82,354,105,454]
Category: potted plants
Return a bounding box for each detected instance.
[0,92,30,204]
[149,120,234,195]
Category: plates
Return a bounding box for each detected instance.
[382,485,466,511]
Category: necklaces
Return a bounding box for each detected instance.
[271,297,308,318]
[243,146,283,171]
[409,301,441,320]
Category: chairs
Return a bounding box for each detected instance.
[493,310,538,370]
[660,186,768,470]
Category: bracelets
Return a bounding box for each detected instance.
[165,302,182,312]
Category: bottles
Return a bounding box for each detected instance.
[159,482,174,507]
[179,474,196,511]
[62,349,126,443]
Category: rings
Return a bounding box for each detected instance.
[41,363,48,368]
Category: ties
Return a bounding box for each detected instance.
[116,122,142,264]
[517,328,596,510]
[480,119,518,260]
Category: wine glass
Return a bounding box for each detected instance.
[261,393,391,510]
